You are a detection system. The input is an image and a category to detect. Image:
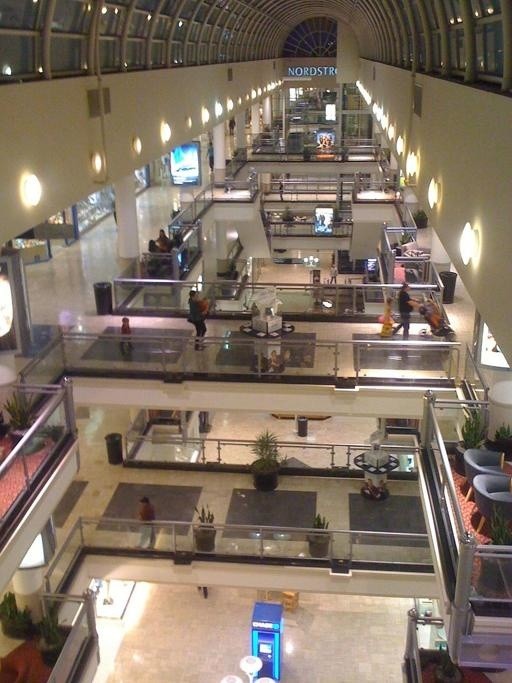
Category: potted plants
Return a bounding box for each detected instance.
[192,505,216,551]
[3,387,38,455]
[474,505,512,598]
[38,614,65,668]
[492,422,512,461]
[454,412,488,476]
[306,513,330,557]
[249,429,281,491]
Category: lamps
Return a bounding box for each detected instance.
[427,177,441,209]
[20,173,42,209]
[459,221,480,267]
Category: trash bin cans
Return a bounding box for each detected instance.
[298,416,308,437]
[93,282,112,315]
[439,271,457,304]
[105,433,123,464]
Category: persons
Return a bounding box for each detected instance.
[197,585,209,598]
[186,288,207,350]
[206,141,214,171]
[196,293,210,313]
[266,349,281,373]
[132,495,157,550]
[380,296,395,337]
[120,317,134,350]
[315,213,334,234]
[157,228,171,242]
[391,281,423,338]
[148,239,168,278]
[223,176,238,192]
[329,263,339,285]
[229,115,236,135]
[280,180,285,200]
[247,165,258,197]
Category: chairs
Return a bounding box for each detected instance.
[463,449,511,502]
[472,474,512,532]
